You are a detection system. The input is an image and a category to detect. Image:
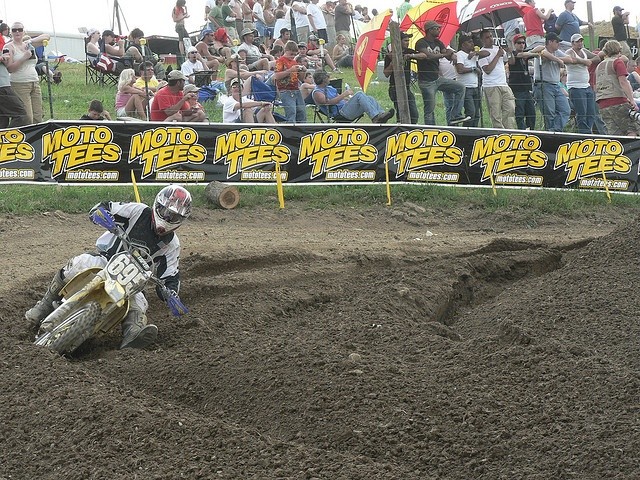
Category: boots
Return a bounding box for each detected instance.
[25,268,67,326]
[120,309,158,350]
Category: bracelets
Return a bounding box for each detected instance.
[412,52,415,58]
[260,99,264,107]
[340,91,346,98]
[471,66,475,73]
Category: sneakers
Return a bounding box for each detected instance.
[54,72,61,83]
[372,112,389,122]
[388,108,395,119]
[450,115,471,123]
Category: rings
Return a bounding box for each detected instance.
[192,93,195,94]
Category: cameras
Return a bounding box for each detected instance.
[294,65,303,71]
[629,106,640,121]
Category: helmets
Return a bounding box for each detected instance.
[151,185,192,236]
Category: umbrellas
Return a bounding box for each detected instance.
[457,0,530,52]
[397,0,458,72]
[353,9,406,95]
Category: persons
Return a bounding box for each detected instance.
[100,30,144,75]
[297,43,309,67]
[611,5,632,61]
[206,0,351,42]
[454,35,491,127]
[223,76,276,123]
[363,7,370,22]
[523,0,553,48]
[115,67,150,121]
[182,43,217,99]
[533,32,571,131]
[300,70,316,101]
[23,185,194,351]
[353,4,364,21]
[333,34,356,69]
[312,69,395,125]
[507,34,534,128]
[238,29,276,71]
[565,35,601,133]
[596,43,637,137]
[632,63,640,86]
[86,28,101,71]
[185,81,207,123]
[154,68,191,122]
[225,53,271,96]
[556,1,592,54]
[307,33,343,74]
[83,101,111,121]
[5,23,46,124]
[126,28,158,65]
[412,18,469,124]
[27,29,62,85]
[2,23,13,48]
[275,41,308,125]
[477,30,507,129]
[171,0,192,55]
[385,29,429,124]
[137,58,158,96]
[0,51,26,126]
[195,29,224,81]
[275,28,293,52]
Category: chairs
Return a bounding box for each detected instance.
[250,77,287,123]
[230,47,269,78]
[83,36,128,86]
[37,55,67,84]
[312,78,364,123]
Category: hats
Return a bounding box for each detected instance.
[114,36,127,43]
[512,34,526,42]
[240,28,254,36]
[183,84,201,93]
[424,21,442,30]
[102,30,118,38]
[613,6,624,11]
[297,42,307,47]
[565,0,576,4]
[402,34,413,39]
[200,29,213,41]
[309,35,319,40]
[230,77,244,86]
[87,28,99,36]
[571,34,584,42]
[168,69,190,81]
[546,32,562,42]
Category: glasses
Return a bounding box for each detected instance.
[232,85,239,88]
[515,41,525,44]
[12,28,23,32]
[191,52,197,54]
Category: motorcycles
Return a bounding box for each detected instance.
[33,204,186,358]
